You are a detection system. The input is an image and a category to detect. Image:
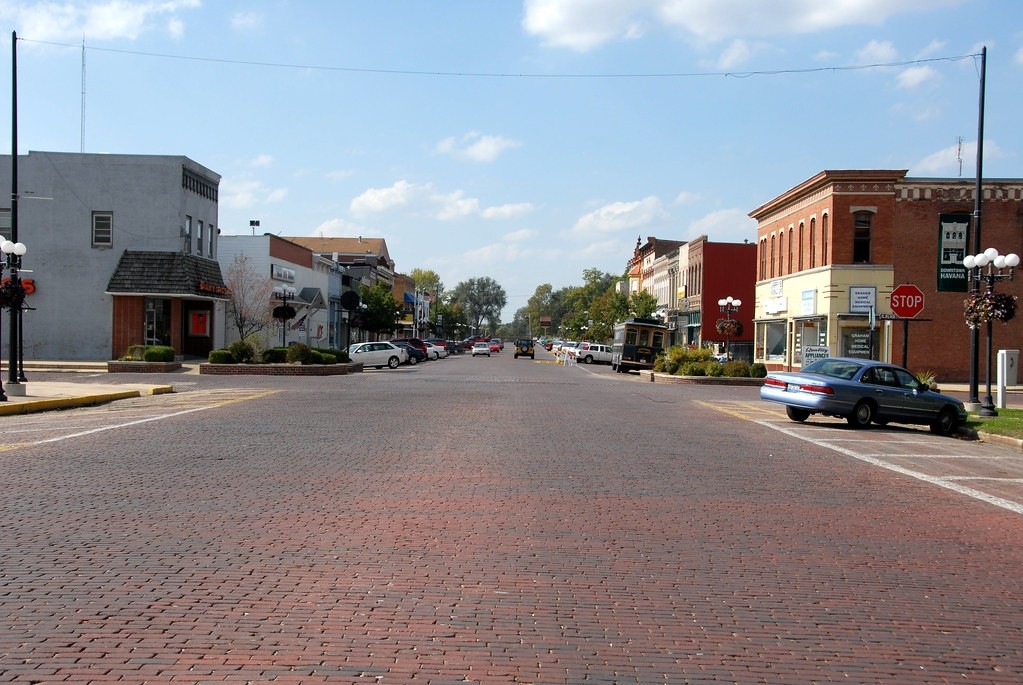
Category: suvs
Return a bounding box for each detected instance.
[514,339,535,360]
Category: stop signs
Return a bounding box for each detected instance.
[889,283,925,319]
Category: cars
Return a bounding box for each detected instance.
[342,341,406,369]
[760,356,968,436]
[533,336,596,355]
[462,336,489,350]
[472,342,490,357]
[389,338,463,364]
[489,338,505,352]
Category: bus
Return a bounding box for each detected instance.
[612,317,669,373]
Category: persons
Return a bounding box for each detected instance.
[688,340,695,350]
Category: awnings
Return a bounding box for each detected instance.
[405,292,422,305]
[683,323,702,327]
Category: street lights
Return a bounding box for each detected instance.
[0,236,26,400]
[716,296,741,362]
[964,249,1021,417]
[581,326,588,340]
[273,284,297,348]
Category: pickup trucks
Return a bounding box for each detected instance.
[570,345,613,364]
